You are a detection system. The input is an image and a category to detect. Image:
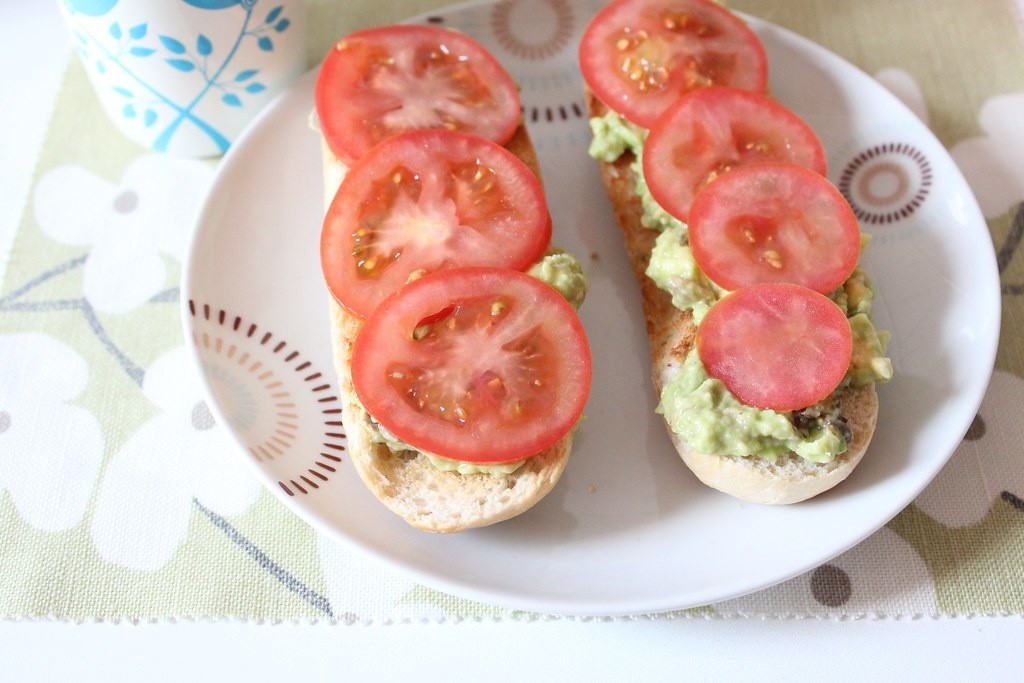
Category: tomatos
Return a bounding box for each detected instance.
[314,0,862,464]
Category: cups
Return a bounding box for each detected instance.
[71,1,308,163]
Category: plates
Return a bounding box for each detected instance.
[181,0,1006,617]
